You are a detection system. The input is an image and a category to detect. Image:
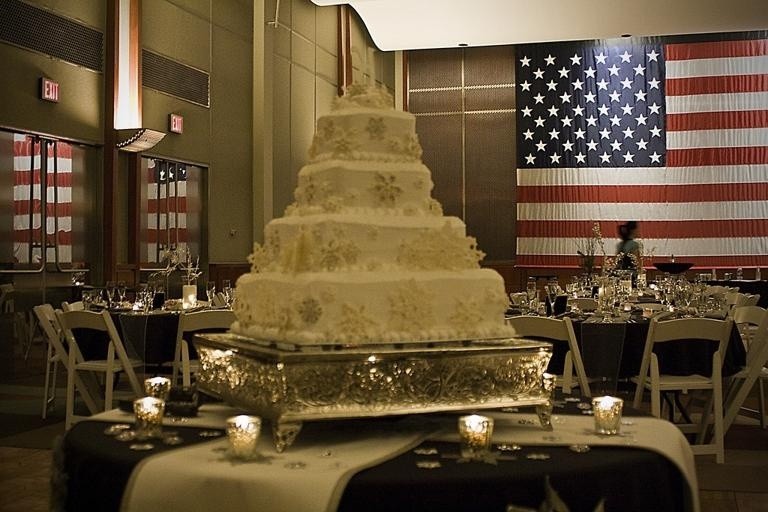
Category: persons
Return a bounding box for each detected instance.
[614,222,644,267]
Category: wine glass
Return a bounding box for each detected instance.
[205,281,216,310]
[509,256,722,323]
[117,281,127,310]
[133,286,157,315]
[82,289,103,312]
[105,281,117,311]
[222,280,233,311]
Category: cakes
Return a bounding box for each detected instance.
[228,81,516,348]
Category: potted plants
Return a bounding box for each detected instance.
[571,235,598,283]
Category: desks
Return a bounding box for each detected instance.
[695,278,767,309]
[74,304,227,390]
[50,390,702,510]
[514,285,733,423]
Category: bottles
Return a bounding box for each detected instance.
[755,267,761,280]
[694,272,712,281]
[723,270,744,281]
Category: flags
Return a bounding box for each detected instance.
[146,157,189,266]
[13,134,75,265]
[517,29,767,269]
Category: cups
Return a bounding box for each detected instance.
[458,414,495,459]
[132,397,166,438]
[226,415,262,461]
[144,377,171,401]
[592,396,624,436]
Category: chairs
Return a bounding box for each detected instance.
[708,305,768,447]
[170,308,236,389]
[627,314,732,465]
[502,314,600,400]
[61,301,84,311]
[34,302,100,418]
[53,308,145,432]
